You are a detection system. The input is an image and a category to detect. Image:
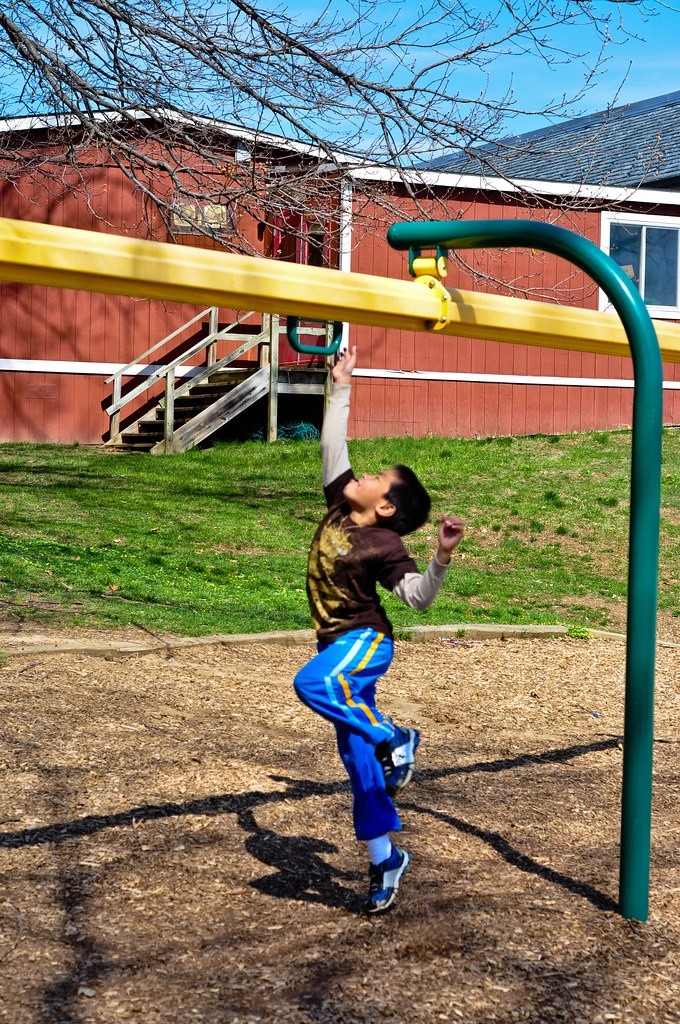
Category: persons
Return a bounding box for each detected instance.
[293,346,465,913]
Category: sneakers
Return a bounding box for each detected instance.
[363,843,413,914]
[374,726,420,797]
